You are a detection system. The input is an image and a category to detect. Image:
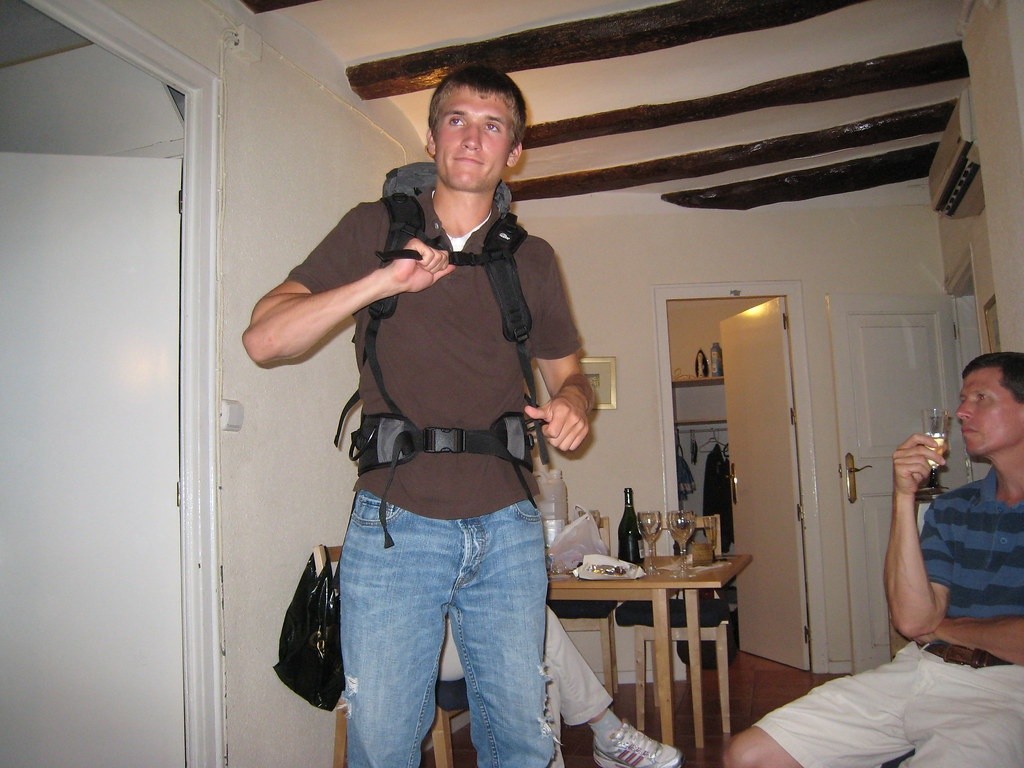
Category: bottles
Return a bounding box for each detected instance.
[617,488,645,566]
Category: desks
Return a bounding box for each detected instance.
[549,554,753,746]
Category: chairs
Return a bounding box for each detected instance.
[558,516,619,713]
[314,545,465,768]
[634,514,731,733]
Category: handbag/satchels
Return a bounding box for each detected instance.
[273,544,345,711]
[544,504,609,574]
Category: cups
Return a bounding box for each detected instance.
[538,468,568,571]
[691,544,713,566]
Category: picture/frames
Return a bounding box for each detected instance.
[579,357,617,410]
[984,294,1000,353]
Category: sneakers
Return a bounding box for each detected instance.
[592,717,684,768]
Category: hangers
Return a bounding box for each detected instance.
[690,430,698,465]
[699,428,729,457]
[676,429,683,459]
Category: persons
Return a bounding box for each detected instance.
[438,604,686,768]
[243,68,594,768]
[723,351,1023,768]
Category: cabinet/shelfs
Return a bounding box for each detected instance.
[672,376,726,424]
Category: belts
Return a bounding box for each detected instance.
[916,641,1013,669]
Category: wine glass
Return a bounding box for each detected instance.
[667,510,696,579]
[637,511,663,576]
[912,408,953,499]
[702,516,722,564]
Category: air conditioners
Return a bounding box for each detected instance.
[929,84,984,218]
